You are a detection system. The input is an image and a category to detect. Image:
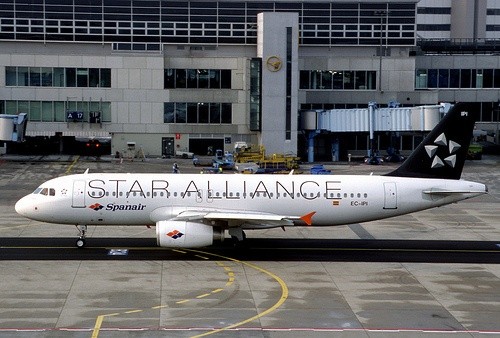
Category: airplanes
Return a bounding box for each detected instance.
[13,99,489,251]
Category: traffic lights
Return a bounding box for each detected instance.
[86,143,90,147]
[95,143,100,148]
